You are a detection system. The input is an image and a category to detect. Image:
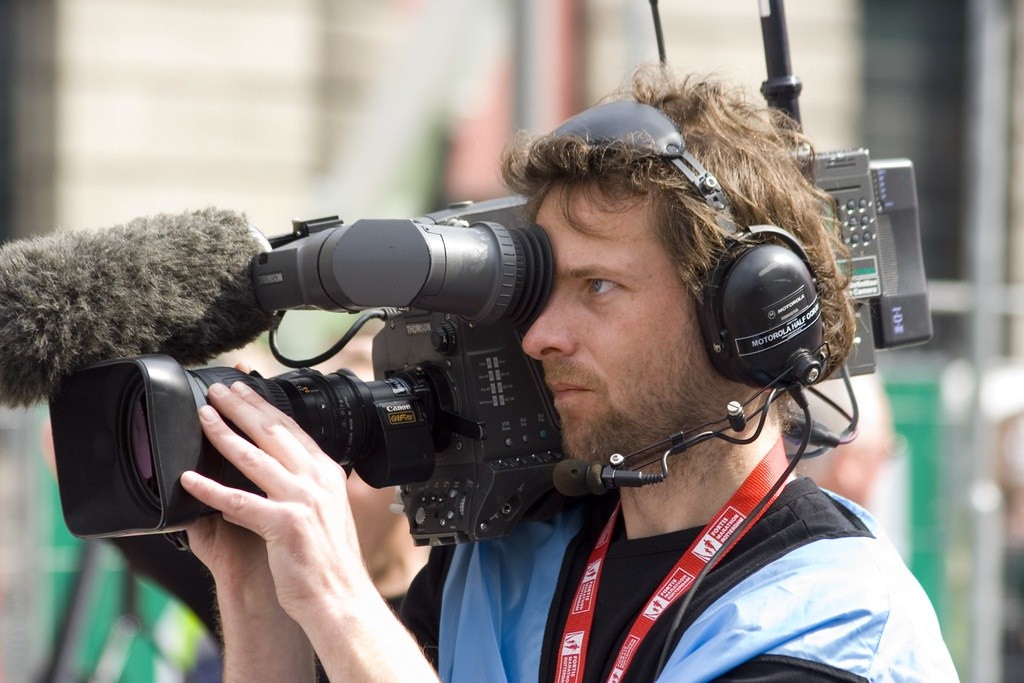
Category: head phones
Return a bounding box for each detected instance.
[551,101,830,391]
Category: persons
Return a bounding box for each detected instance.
[174,74,965,683]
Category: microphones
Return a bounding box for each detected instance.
[551,458,663,497]
[0,208,279,411]
